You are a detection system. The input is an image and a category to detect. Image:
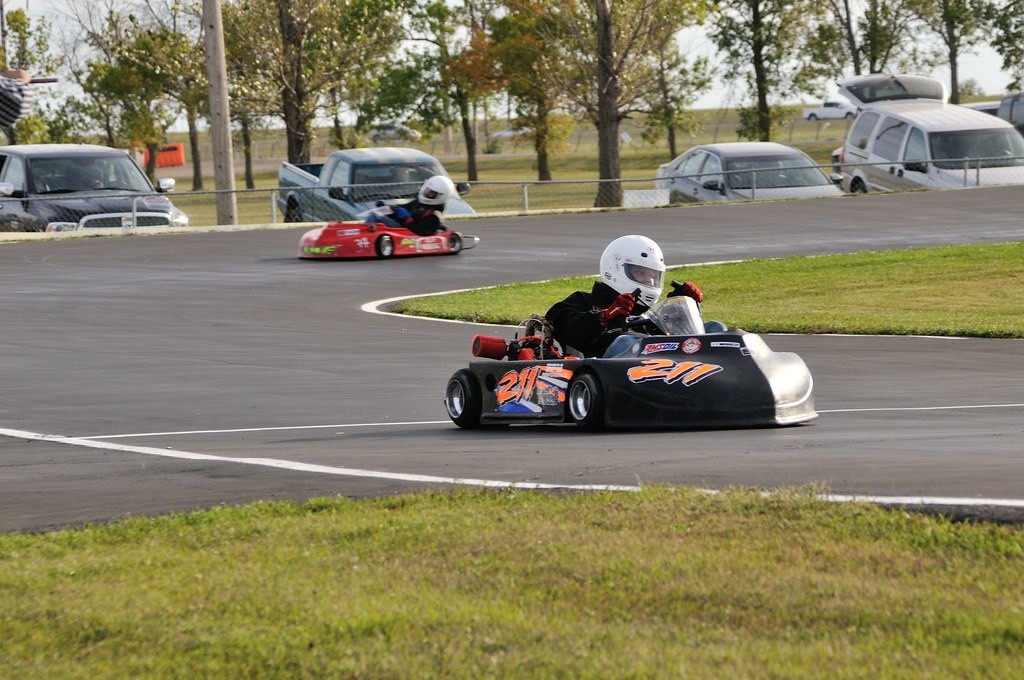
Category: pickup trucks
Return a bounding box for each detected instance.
[802,98,858,123]
[275,146,477,222]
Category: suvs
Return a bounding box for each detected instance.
[835,73,1024,195]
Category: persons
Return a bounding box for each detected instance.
[360,175,455,240]
[540,232,705,360]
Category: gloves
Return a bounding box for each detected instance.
[601,293,635,322]
[392,205,411,219]
[376,201,384,207]
[668,282,703,303]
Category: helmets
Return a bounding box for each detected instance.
[600,233,665,307]
[418,176,452,205]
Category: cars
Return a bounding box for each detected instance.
[488,123,537,143]
[0,143,192,234]
[368,122,423,145]
[655,141,846,207]
